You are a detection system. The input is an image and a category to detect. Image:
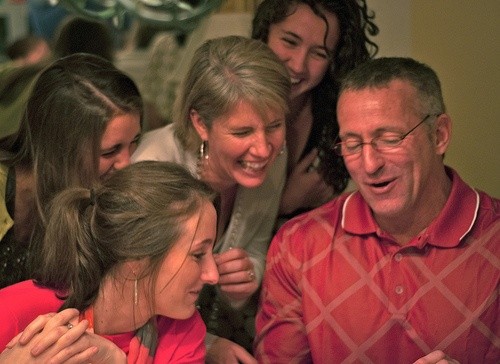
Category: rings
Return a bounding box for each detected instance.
[66,324,72,329]
[248,270,254,278]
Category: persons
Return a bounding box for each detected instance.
[255,57,500,363]
[0,1,290,363]
[252,0,379,237]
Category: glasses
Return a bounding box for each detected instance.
[331,113,442,155]
